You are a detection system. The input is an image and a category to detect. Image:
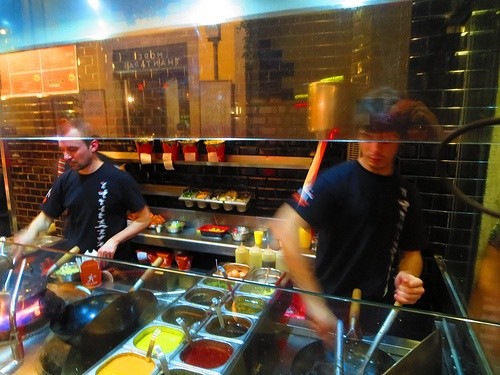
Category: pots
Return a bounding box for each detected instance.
[0,246,80,317]
[291,289,396,375]
[49,257,164,375]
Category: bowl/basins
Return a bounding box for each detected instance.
[197,224,230,238]
[165,221,184,234]
[230,227,251,241]
[54,262,80,283]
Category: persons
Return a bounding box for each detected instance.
[477,220,500,375]
[270,87,425,351]
[9,120,152,273]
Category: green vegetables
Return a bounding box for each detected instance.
[183,187,197,198]
[205,281,233,290]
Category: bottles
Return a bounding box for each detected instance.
[254,229,263,247]
[249,243,262,267]
[276,248,289,273]
[235,242,249,266]
[262,245,276,269]
